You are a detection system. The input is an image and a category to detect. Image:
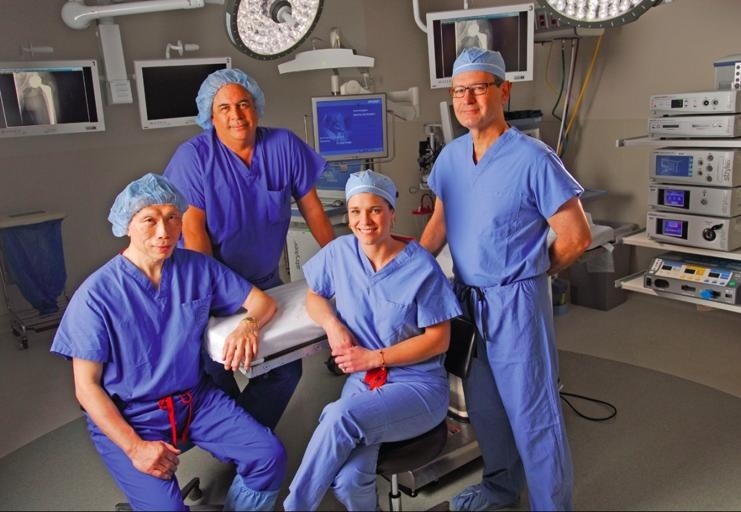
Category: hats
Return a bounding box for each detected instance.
[452,46,506,80]
[194,68,265,129]
[344,169,397,211]
[107,172,188,238]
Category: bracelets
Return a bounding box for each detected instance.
[380,349,385,371]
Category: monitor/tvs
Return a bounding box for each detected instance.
[315,160,365,192]
[425,3,537,90]
[309,93,391,164]
[1,60,107,138]
[133,56,233,131]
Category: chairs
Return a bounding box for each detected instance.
[116,433,204,512]
[376,315,476,511]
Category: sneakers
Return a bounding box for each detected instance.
[448,481,522,512]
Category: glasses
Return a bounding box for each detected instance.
[449,82,502,99]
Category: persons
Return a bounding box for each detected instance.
[419,46,593,512]
[49,172,288,512]
[283,170,463,512]
[161,68,337,432]
